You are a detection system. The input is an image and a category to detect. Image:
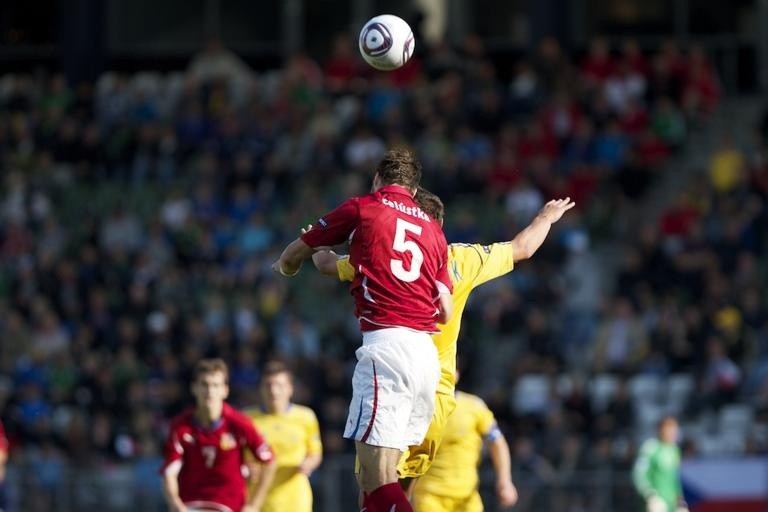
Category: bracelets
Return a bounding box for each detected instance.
[279,262,300,278]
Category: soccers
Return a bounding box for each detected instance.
[360,15,413,68]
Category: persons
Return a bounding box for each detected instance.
[237,361,323,511]
[271,146,454,509]
[0,18,766,512]
[403,364,520,510]
[161,359,277,511]
[301,184,575,511]
[632,415,689,512]
